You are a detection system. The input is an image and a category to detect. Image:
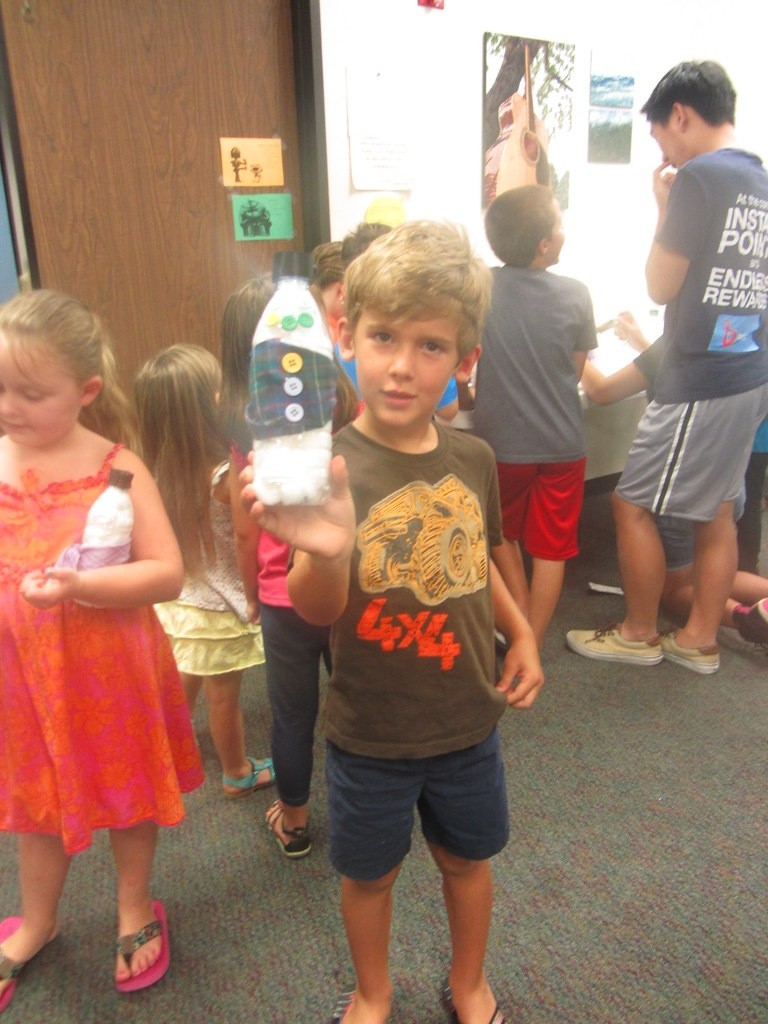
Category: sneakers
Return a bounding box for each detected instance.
[566,620,664,665]
[657,623,720,674]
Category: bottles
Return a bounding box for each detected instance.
[71,469,136,608]
[247,249,349,504]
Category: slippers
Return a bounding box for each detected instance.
[332,984,397,1024]
[443,977,507,1024]
[0,917,24,1013]
[114,900,170,991]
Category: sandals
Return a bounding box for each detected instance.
[223,756,277,799]
[264,799,312,858]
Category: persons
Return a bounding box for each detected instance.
[134,343,280,801]
[581,309,767,654]
[463,182,597,652]
[0,287,206,1014]
[221,275,363,856]
[328,221,459,419]
[570,61,767,676]
[306,237,349,343]
[236,218,545,1024]
